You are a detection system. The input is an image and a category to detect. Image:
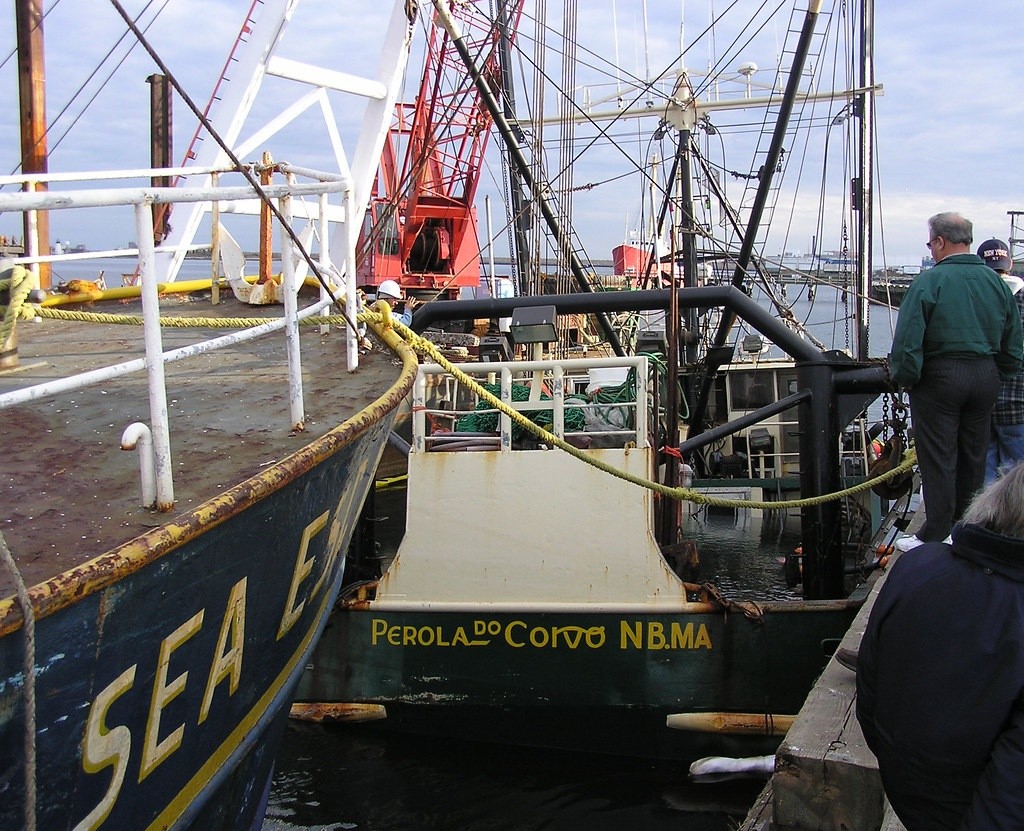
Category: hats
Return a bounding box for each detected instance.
[977,237,1010,270]
[376,279,403,300]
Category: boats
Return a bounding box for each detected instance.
[0,0,419,831]
[289,0,896,719]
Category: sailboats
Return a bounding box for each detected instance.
[612,152,682,279]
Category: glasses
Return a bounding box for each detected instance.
[926,239,939,249]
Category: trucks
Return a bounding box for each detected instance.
[896,267,921,275]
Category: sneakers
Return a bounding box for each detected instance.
[896,534,953,552]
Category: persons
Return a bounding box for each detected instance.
[976,238,1024,491]
[888,213,1024,552]
[376,280,418,328]
[854,462,1024,831]
